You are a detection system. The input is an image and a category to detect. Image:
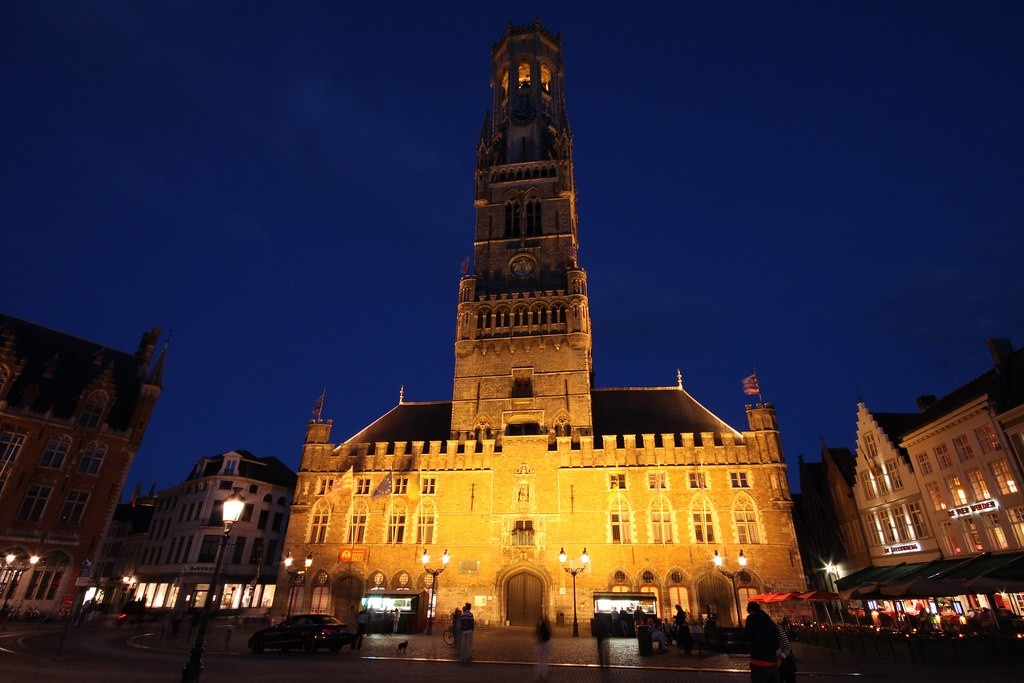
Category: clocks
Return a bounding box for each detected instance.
[509,254,537,277]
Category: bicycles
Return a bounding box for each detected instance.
[442,618,456,646]
[3,603,40,623]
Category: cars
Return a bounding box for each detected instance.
[247,613,358,656]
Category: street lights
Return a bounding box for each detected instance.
[284,551,314,620]
[421,548,450,635]
[713,549,746,627]
[0,553,41,616]
[178,485,246,683]
[559,547,590,637]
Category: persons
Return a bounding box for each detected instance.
[608,602,645,637]
[346,604,371,653]
[534,613,553,682]
[366,603,399,637]
[646,619,669,652]
[451,601,474,662]
[672,604,685,644]
[741,602,797,683]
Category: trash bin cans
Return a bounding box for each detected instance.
[636,625,653,654]
[557,612,564,625]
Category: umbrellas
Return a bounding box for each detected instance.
[749,590,861,628]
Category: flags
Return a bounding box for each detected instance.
[341,469,351,493]
[372,472,392,498]
[742,374,760,395]
[406,472,422,501]
[312,394,322,415]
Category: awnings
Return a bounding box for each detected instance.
[833,550,1024,598]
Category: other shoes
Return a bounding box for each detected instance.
[466,656,474,661]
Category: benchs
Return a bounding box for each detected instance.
[646,623,752,654]
